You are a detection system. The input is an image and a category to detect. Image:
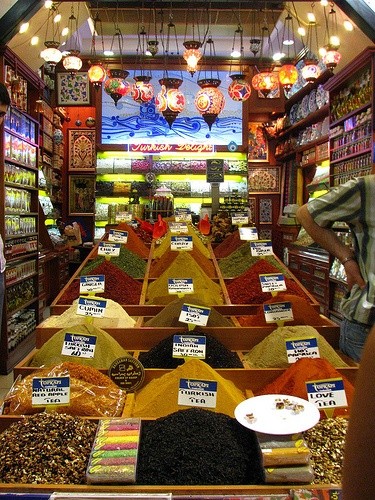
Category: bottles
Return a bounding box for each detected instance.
[152,182,174,219]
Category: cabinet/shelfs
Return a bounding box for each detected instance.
[0,44,69,380]
[278,45,375,329]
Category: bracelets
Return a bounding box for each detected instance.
[342,255,356,264]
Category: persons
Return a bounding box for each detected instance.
[296,174,375,500]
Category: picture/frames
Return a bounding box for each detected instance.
[66,172,96,217]
[248,166,282,195]
[248,121,270,162]
[54,69,92,107]
[67,128,96,171]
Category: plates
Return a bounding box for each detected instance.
[297,116,329,145]
[234,394,321,435]
[289,84,329,125]
[284,60,326,98]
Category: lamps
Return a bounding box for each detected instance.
[19,0,356,132]
